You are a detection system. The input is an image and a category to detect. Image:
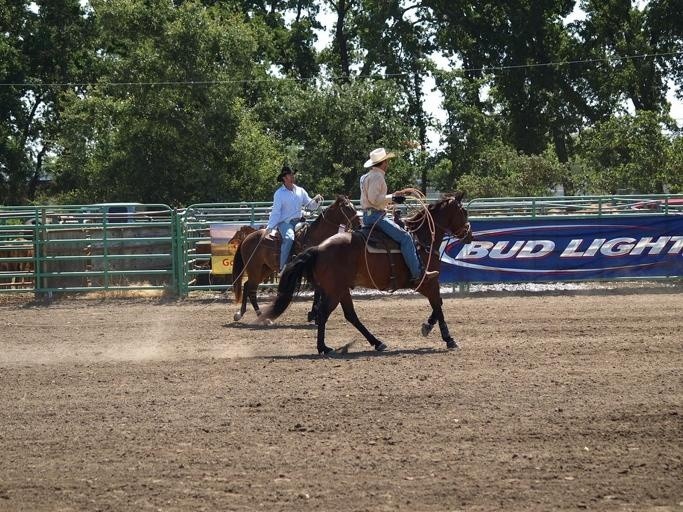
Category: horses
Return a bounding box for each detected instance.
[0,237,35,291]
[251,189,473,357]
[232,192,362,327]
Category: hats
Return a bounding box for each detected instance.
[364,148,395,168]
[278,166,298,182]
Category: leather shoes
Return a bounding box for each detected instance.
[414,271,439,285]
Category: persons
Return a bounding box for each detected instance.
[266,164,321,272]
[359,147,440,286]
